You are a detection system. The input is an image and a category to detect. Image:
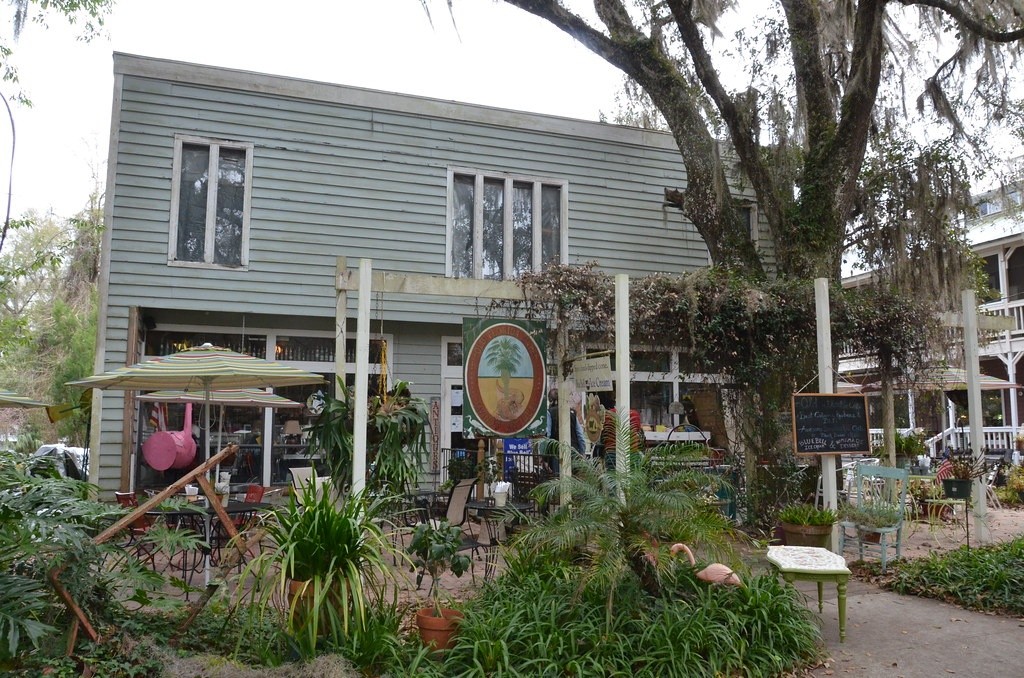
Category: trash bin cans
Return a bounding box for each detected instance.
[705,467,738,520]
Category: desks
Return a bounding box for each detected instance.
[146,500,270,579]
[462,502,534,587]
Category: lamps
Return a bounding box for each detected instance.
[282,420,303,444]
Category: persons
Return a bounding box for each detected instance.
[543,388,587,473]
[603,407,642,473]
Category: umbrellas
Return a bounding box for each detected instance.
[0,386,52,409]
[63,342,324,590]
[138,389,305,490]
[870,363,1024,452]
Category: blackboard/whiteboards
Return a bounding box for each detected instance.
[791,392,872,456]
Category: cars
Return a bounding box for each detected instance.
[29,443,91,481]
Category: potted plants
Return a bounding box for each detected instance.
[782,504,838,547]
[249,485,386,639]
[411,525,473,664]
[941,448,987,498]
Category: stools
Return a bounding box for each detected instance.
[764,545,854,643]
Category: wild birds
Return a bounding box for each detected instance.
[670,543,742,586]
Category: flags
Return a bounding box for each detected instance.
[936,448,955,485]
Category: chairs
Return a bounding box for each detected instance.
[114,477,506,572]
[838,464,909,575]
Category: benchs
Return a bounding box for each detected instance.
[908,475,966,523]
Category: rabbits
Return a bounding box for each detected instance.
[493,379,525,420]
